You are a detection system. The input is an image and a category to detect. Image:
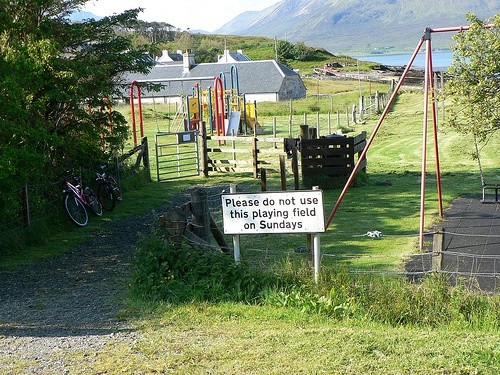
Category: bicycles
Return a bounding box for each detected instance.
[56,169,103,227]
[95,162,122,212]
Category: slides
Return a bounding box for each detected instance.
[225,108,242,137]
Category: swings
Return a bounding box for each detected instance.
[459,32,500,204]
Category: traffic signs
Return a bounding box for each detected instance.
[221,189,326,235]
